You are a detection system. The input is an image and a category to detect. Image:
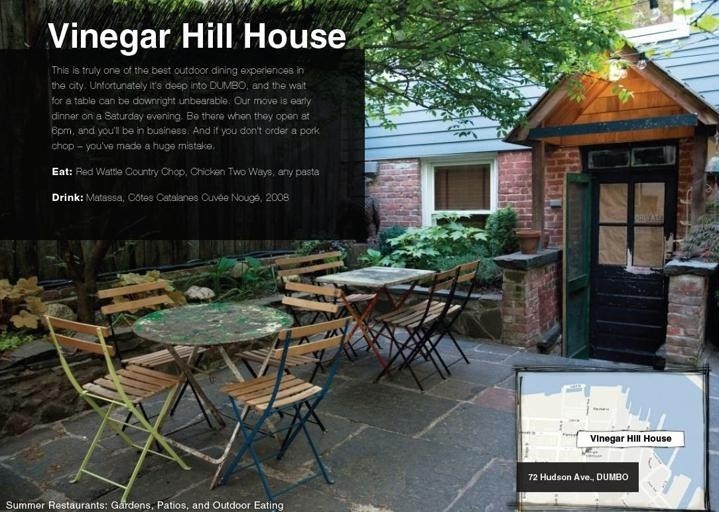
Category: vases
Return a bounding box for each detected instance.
[515,228,541,253]
